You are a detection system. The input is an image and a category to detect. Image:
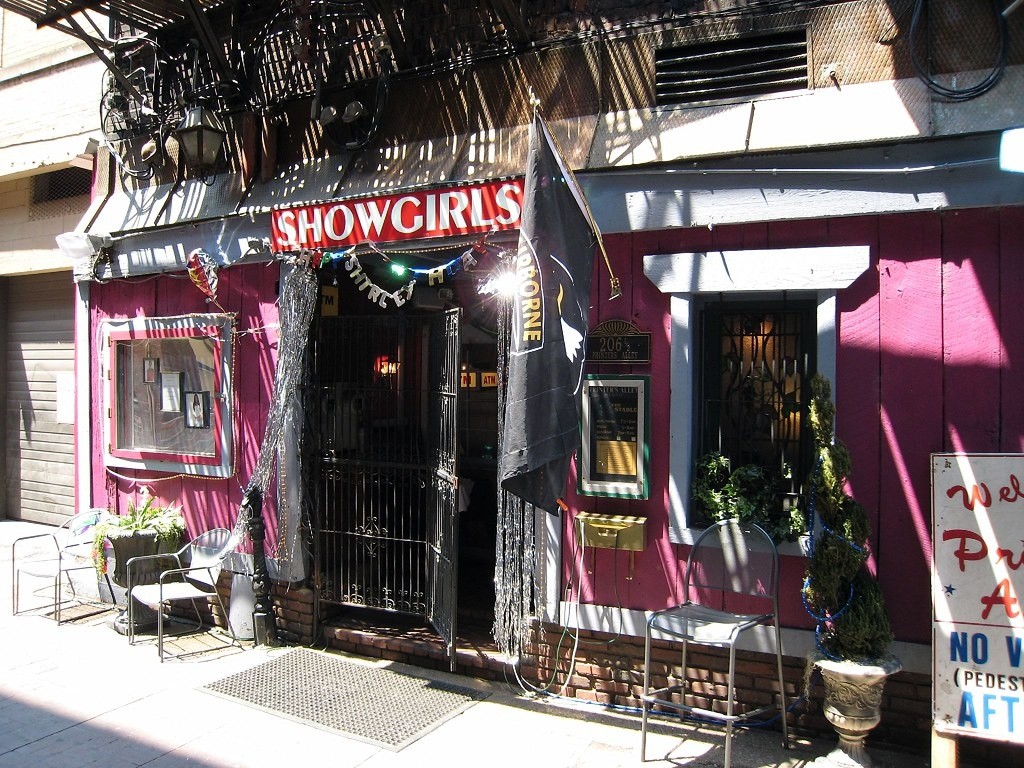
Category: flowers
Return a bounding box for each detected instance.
[93,493,187,580]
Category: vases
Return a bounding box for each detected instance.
[107,530,166,624]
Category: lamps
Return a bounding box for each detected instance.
[173,98,230,187]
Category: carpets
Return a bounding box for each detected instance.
[197,646,493,752]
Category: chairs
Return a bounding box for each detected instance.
[12,509,116,626]
[127,527,243,662]
[641,519,788,768]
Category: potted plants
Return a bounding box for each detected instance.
[804,373,902,768]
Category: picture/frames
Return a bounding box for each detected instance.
[142,357,159,384]
[184,390,210,428]
